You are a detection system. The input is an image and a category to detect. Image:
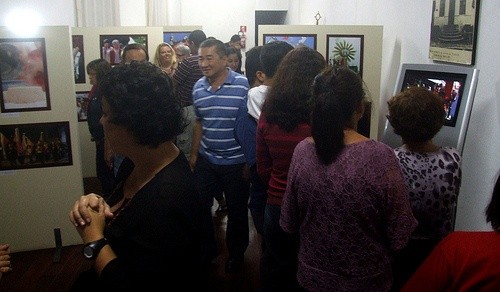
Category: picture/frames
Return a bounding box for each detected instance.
[76,91,101,122]
[263,33,318,53]
[99,32,149,66]
[0,120,73,170]
[325,33,365,74]
[0,37,52,113]
[163,30,193,45]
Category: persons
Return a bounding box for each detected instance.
[121,29,500,292]
[69,60,221,292]
[0,243,10,273]
[0,39,121,167]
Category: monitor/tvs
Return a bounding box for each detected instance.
[400,68,468,128]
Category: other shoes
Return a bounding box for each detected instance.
[224,255,244,272]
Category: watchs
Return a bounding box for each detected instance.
[82,238,109,260]
